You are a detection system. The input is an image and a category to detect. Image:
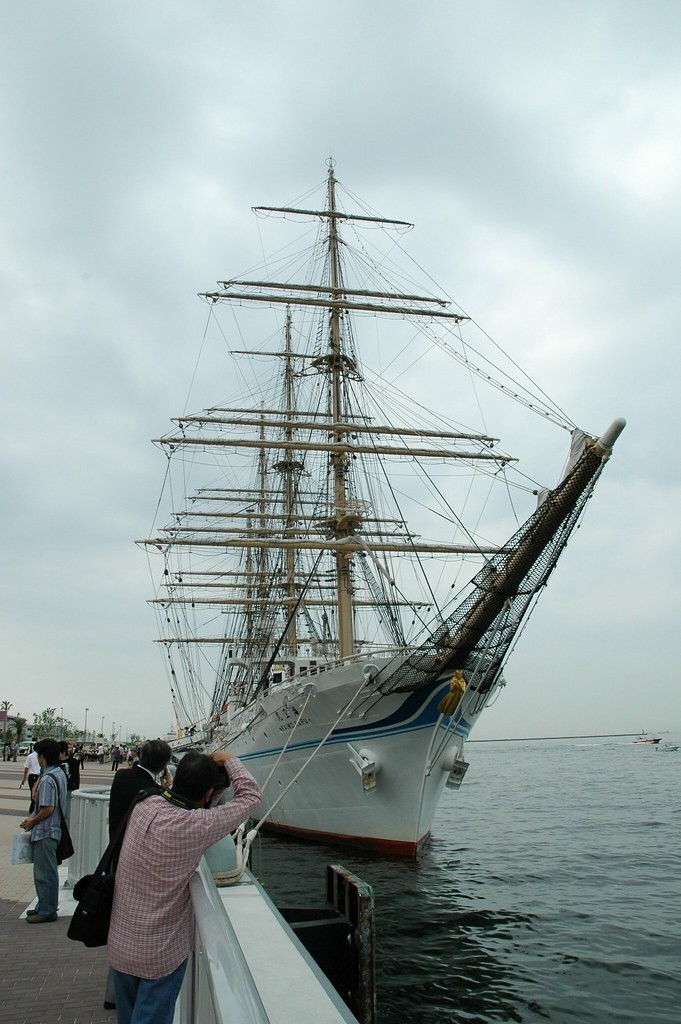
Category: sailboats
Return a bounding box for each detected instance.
[136,154,628,862]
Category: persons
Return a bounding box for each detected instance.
[21,748,41,815]
[21,738,69,924]
[57,740,81,827]
[106,752,263,1023]
[67,742,143,771]
[104,739,172,1012]
[2,742,19,762]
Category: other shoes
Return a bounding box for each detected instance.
[27,910,38,916]
[26,913,57,923]
[103,1001,116,1009]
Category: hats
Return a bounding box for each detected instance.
[130,748,139,752]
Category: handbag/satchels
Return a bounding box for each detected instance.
[56,821,74,866]
[67,787,160,948]
[12,833,33,864]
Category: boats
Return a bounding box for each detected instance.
[655,742,679,751]
[632,736,662,744]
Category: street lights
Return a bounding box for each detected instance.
[101,717,104,734]
[85,708,90,738]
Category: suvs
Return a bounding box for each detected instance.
[18,741,34,755]
